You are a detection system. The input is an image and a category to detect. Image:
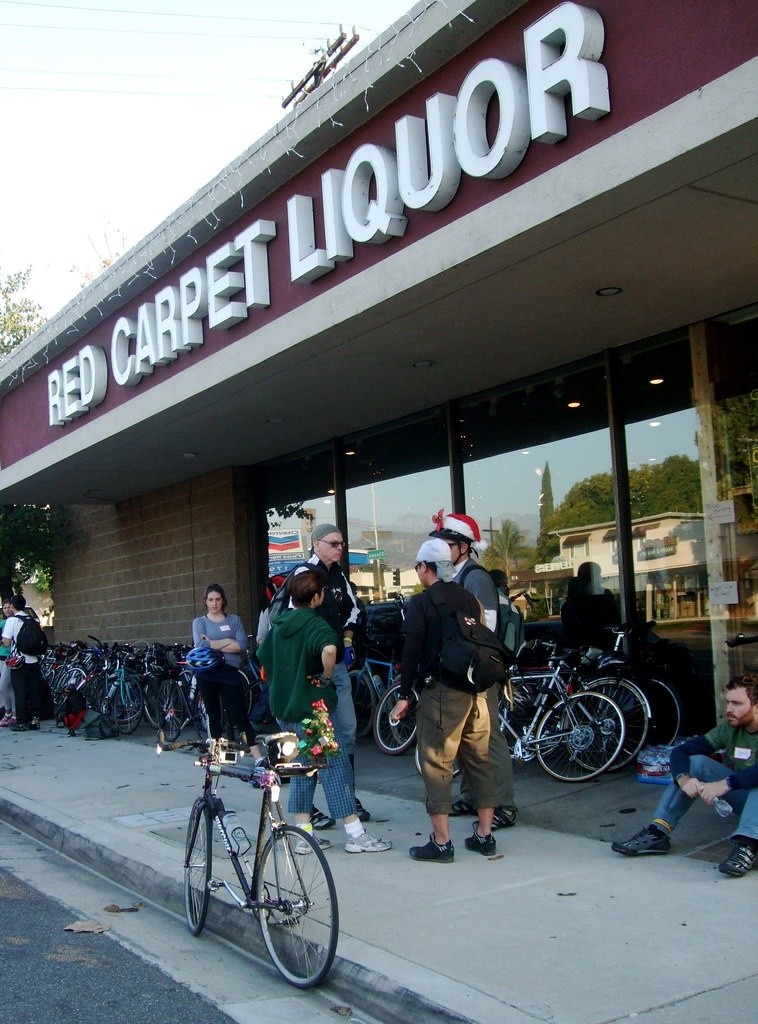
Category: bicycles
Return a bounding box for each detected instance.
[38,634,265,741]
[156,727,338,988]
[348,636,682,784]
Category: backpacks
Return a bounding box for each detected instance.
[425,586,513,694]
[56,688,87,737]
[459,564,526,660]
[11,615,48,656]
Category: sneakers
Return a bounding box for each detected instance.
[355,798,371,822]
[465,821,496,855]
[611,826,669,856]
[448,799,478,816]
[718,840,758,877]
[8,721,40,731]
[294,836,331,854]
[345,828,392,853]
[408,833,454,863]
[310,805,336,830]
[472,809,517,831]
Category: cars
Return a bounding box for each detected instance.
[522,620,692,684]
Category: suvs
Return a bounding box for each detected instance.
[363,598,412,642]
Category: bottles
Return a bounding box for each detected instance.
[699,782,733,817]
[373,674,386,698]
[107,681,117,698]
[218,810,252,854]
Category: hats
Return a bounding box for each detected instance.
[416,538,458,581]
[443,513,481,542]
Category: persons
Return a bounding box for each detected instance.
[560,561,623,654]
[388,545,504,864]
[255,569,393,856]
[192,583,270,774]
[488,568,525,623]
[286,522,372,832]
[428,512,520,834]
[0,594,43,732]
[610,672,758,877]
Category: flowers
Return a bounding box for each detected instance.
[432,507,443,531]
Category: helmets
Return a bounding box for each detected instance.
[186,647,224,672]
[5,656,25,669]
[428,527,473,542]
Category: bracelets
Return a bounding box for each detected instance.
[343,637,353,643]
[397,693,411,701]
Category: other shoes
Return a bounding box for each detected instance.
[0,714,17,726]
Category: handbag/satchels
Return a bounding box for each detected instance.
[82,710,120,741]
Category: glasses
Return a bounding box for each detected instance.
[313,538,346,548]
[414,560,427,573]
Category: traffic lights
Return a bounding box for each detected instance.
[392,568,400,586]
